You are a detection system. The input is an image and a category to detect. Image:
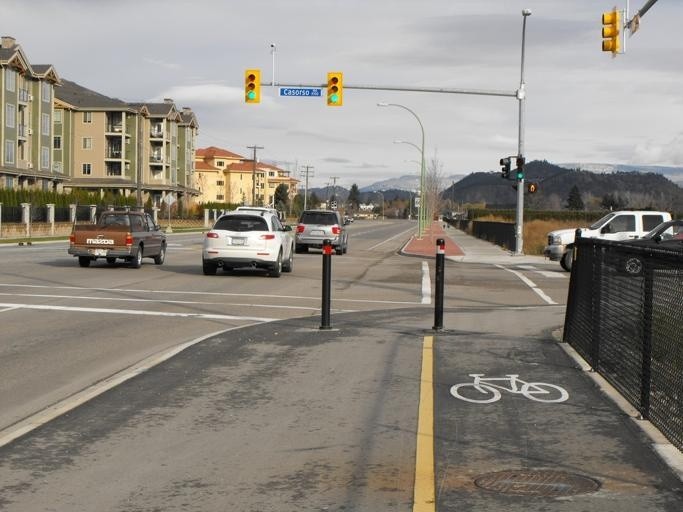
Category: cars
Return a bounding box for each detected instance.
[202,207,294,277]
[612,220,683,275]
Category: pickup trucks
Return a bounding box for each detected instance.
[68,211,167,268]
[543,211,674,275]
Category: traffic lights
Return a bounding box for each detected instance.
[600,9,620,53]
[244,68,259,104]
[529,181,538,193]
[326,70,344,107]
[500,154,524,180]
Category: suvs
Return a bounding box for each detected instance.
[296,208,348,255]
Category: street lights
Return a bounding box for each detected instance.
[377,101,431,238]
[514,8,532,251]
